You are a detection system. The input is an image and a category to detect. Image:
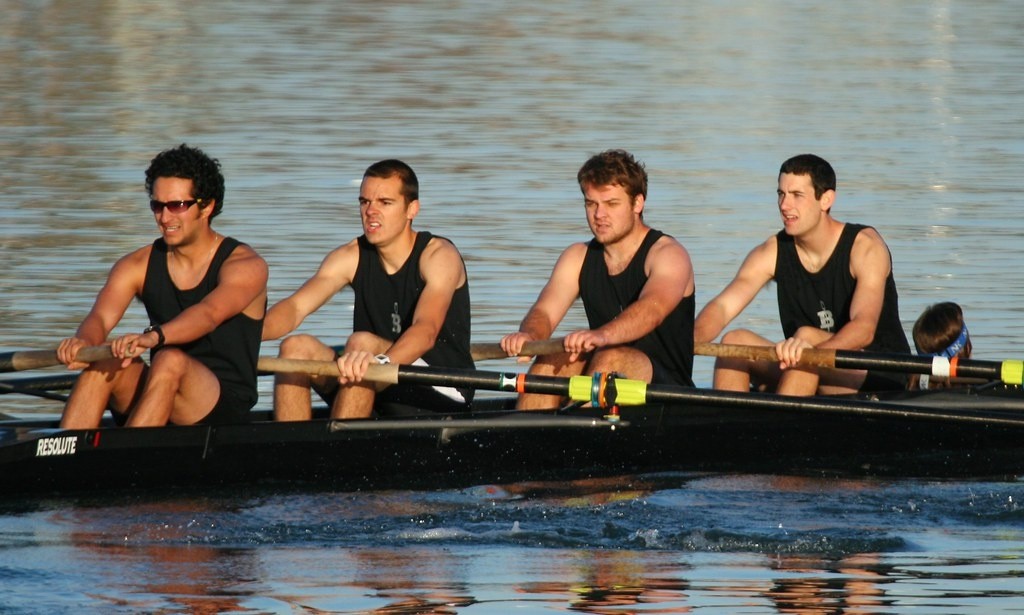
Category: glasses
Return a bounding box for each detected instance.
[149,199,206,213]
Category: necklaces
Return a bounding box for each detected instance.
[170,231,218,288]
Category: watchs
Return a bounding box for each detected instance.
[143,324,166,350]
[374,354,391,365]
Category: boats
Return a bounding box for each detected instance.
[0,381,1024,502]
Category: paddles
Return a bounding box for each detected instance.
[257,356,1024,430]
[693,339,1021,386]
[0,344,135,373]
[254,334,566,376]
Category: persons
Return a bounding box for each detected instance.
[693,154,912,396]
[501,148,695,413]
[57,142,270,432]
[262,159,476,421]
